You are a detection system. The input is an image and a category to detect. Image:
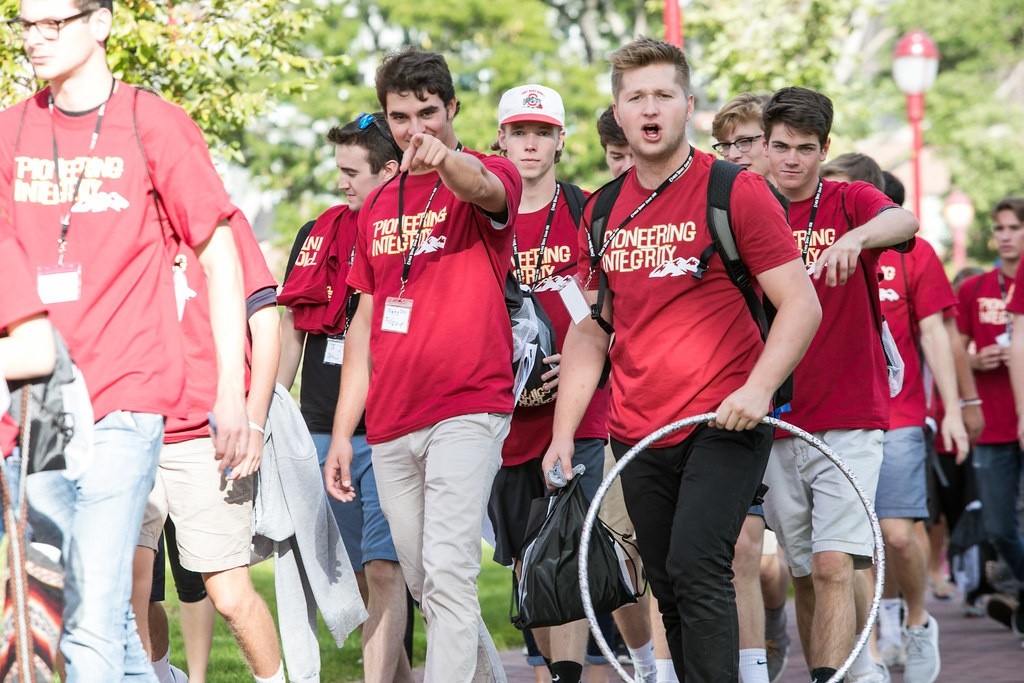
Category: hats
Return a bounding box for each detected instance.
[498,84,565,127]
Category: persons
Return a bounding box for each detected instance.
[0,223,57,380]
[1006,252,1023,452]
[0,0,249,683]
[324,52,522,682]
[133,202,285,683]
[486,84,675,683]
[710,91,982,683]
[757,86,919,683]
[959,196,1024,632]
[277,113,420,682]
[540,36,823,683]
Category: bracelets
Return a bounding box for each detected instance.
[960,400,982,407]
[249,422,264,434]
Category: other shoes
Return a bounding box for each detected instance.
[986,593,1019,630]
[963,591,988,616]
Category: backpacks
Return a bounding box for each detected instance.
[591,159,796,411]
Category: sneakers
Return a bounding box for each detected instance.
[634,661,657,683]
[847,637,890,683]
[765,633,791,682]
[879,598,908,669]
[904,610,941,683]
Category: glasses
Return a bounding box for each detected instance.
[353,112,401,164]
[7,9,96,40]
[712,134,763,156]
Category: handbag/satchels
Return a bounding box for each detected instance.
[511,288,559,407]
[508,466,647,631]
